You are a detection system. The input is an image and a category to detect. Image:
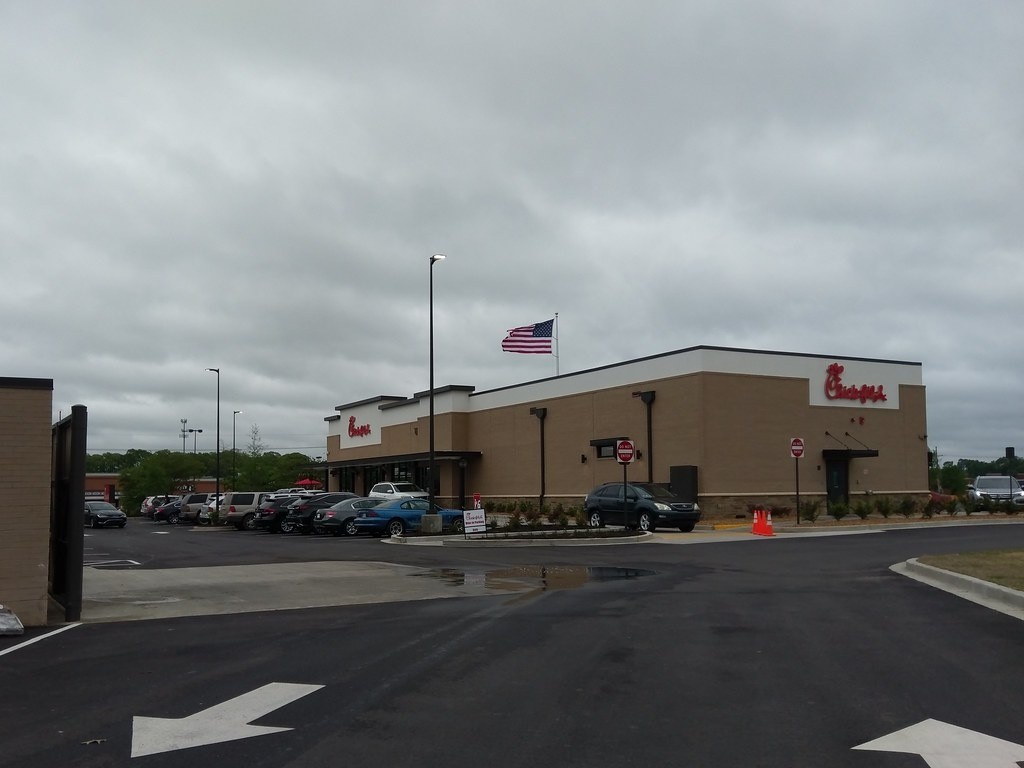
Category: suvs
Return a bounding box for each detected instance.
[368,481,431,507]
[286,490,363,535]
[179,492,304,533]
[583,480,702,531]
[140,495,179,517]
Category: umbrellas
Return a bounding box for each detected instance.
[294,479,320,485]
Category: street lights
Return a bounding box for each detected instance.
[429,252,446,513]
[189,429,203,485]
[233,411,239,491]
[208,368,220,524]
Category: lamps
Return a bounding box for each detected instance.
[329,470,337,478]
[636,450,642,459]
[581,454,587,463]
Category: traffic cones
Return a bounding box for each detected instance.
[750,510,777,536]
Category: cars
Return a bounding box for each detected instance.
[930,491,957,503]
[147,501,173,519]
[277,488,326,493]
[313,497,389,535]
[83,501,127,529]
[354,498,464,537]
[153,500,180,525]
[967,475,1024,507]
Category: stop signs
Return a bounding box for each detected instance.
[791,437,804,457]
[617,441,635,462]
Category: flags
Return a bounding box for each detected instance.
[501,319,554,354]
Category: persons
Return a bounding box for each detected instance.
[153,496,160,522]
[164,494,170,505]
